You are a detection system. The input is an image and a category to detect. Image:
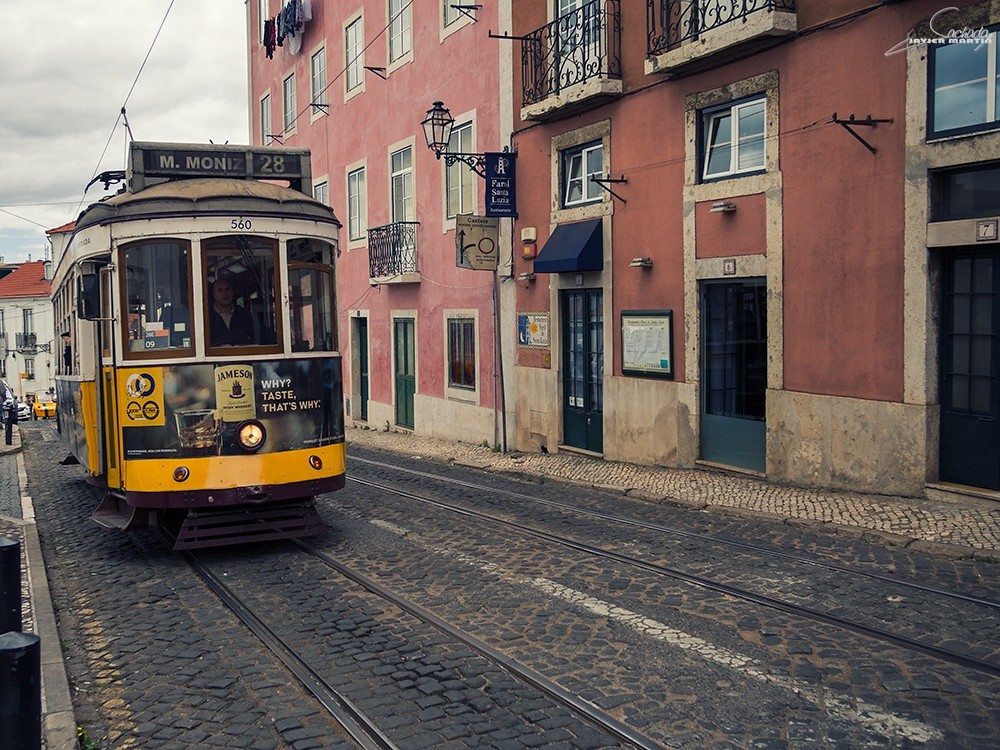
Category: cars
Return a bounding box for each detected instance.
[0,390,58,424]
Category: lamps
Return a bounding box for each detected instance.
[709,202,736,213]
[420,101,518,179]
[630,258,652,267]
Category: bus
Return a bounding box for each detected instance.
[47,139,348,551]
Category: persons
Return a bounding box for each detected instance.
[206,278,276,347]
[132,286,190,346]
[24,394,33,421]
[60,330,71,366]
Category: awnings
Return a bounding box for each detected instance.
[533,217,604,273]
